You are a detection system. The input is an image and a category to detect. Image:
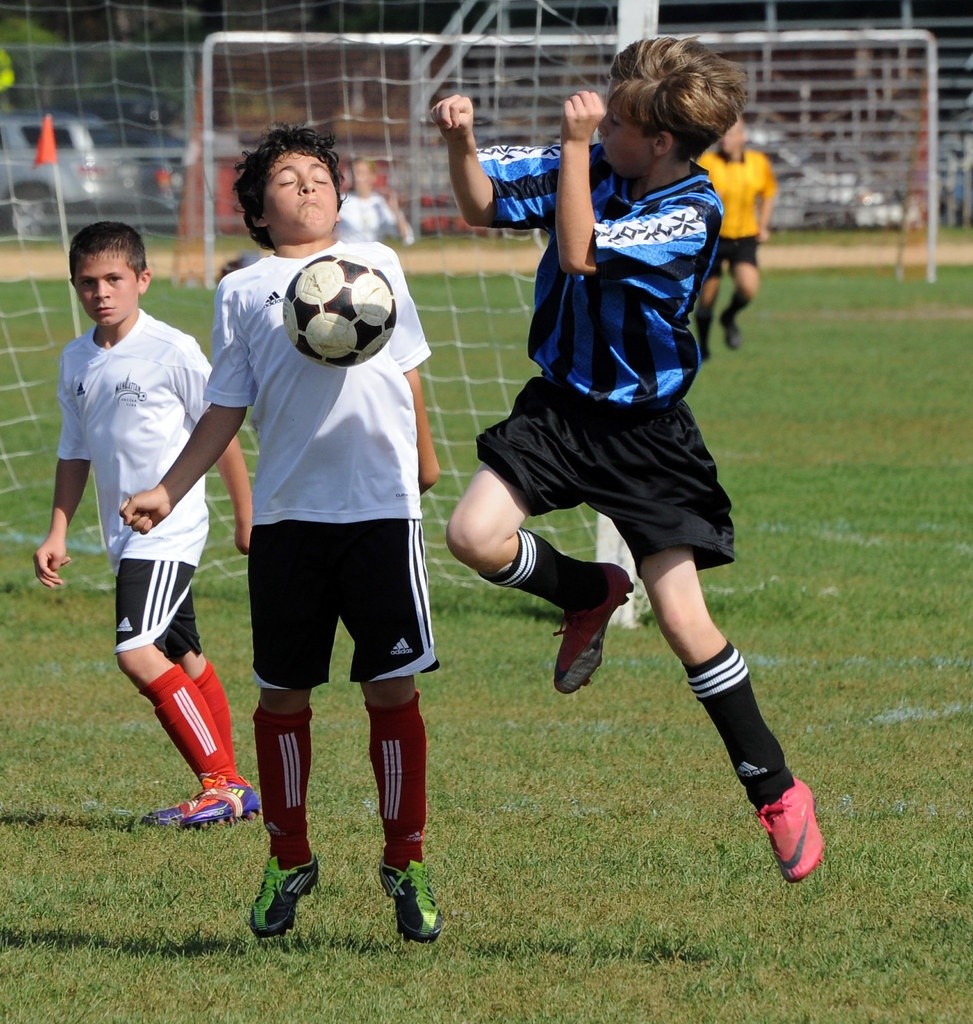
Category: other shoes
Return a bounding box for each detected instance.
[696,337,711,361]
[719,311,741,348]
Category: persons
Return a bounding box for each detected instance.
[336,155,418,245]
[429,38,825,884]
[120,121,444,943]
[697,118,778,364]
[33,221,259,828]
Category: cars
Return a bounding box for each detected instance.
[4,100,973,256]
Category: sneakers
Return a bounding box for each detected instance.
[378,856,443,945]
[179,776,261,828]
[141,791,205,828]
[755,776,825,883]
[554,562,635,694]
[250,851,319,939]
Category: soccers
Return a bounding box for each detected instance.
[279,251,400,370]
